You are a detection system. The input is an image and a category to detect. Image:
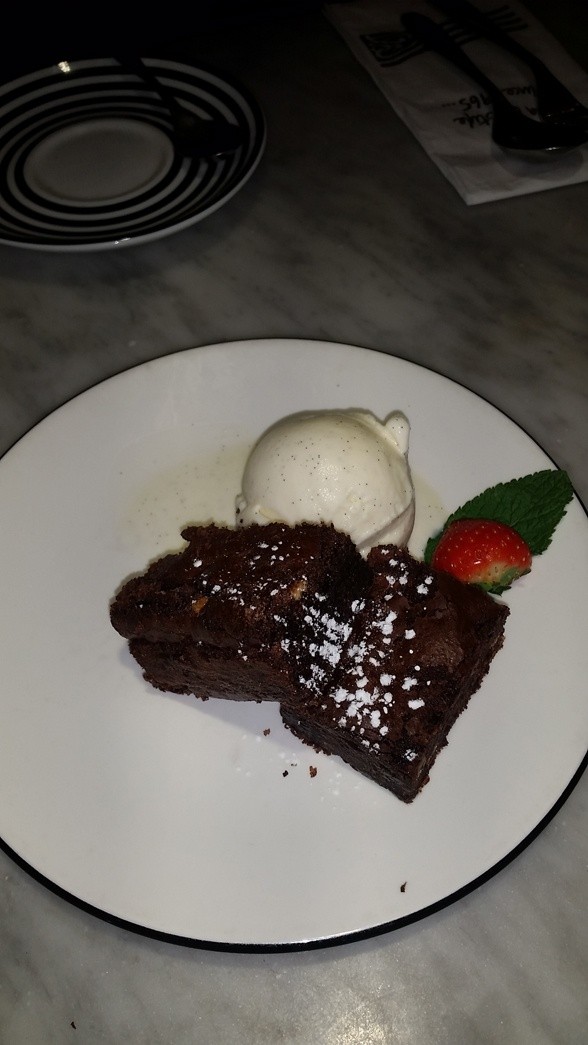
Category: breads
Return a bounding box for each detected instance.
[281,544,510,802]
[108,522,374,704]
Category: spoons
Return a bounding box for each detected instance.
[399,9,576,160]
[109,46,246,161]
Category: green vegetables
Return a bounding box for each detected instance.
[423,468,574,569]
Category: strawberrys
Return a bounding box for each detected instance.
[432,518,533,597]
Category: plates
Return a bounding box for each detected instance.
[0,56,268,254]
[0,336,588,946]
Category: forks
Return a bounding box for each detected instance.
[436,0,588,145]
